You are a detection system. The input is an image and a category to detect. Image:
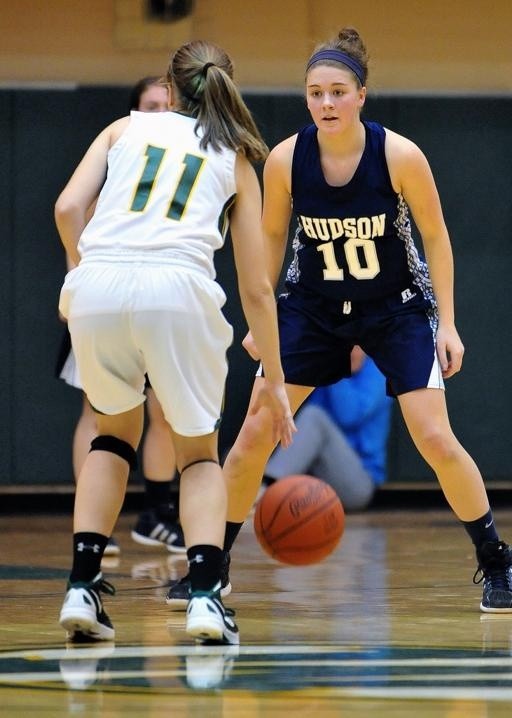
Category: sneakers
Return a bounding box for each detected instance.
[184,592,241,647]
[131,510,188,555]
[165,552,234,612]
[473,539,512,615]
[59,570,116,644]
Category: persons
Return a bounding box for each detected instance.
[52,35,297,646]
[246,334,394,518]
[55,73,209,561]
[58,640,240,716]
[161,30,509,611]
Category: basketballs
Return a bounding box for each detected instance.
[253,475,344,567]
